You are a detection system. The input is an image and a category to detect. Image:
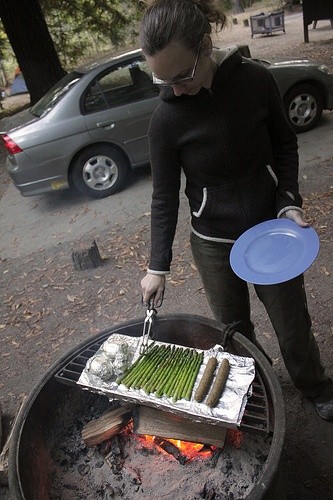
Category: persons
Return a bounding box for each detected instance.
[140,0,333,419]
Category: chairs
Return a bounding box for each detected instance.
[129,65,160,94]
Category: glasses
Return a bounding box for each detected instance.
[153,44,201,87]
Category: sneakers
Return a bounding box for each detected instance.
[316,399,333,420]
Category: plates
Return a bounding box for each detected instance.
[229,218,320,286]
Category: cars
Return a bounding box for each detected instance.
[0,47,332,198]
